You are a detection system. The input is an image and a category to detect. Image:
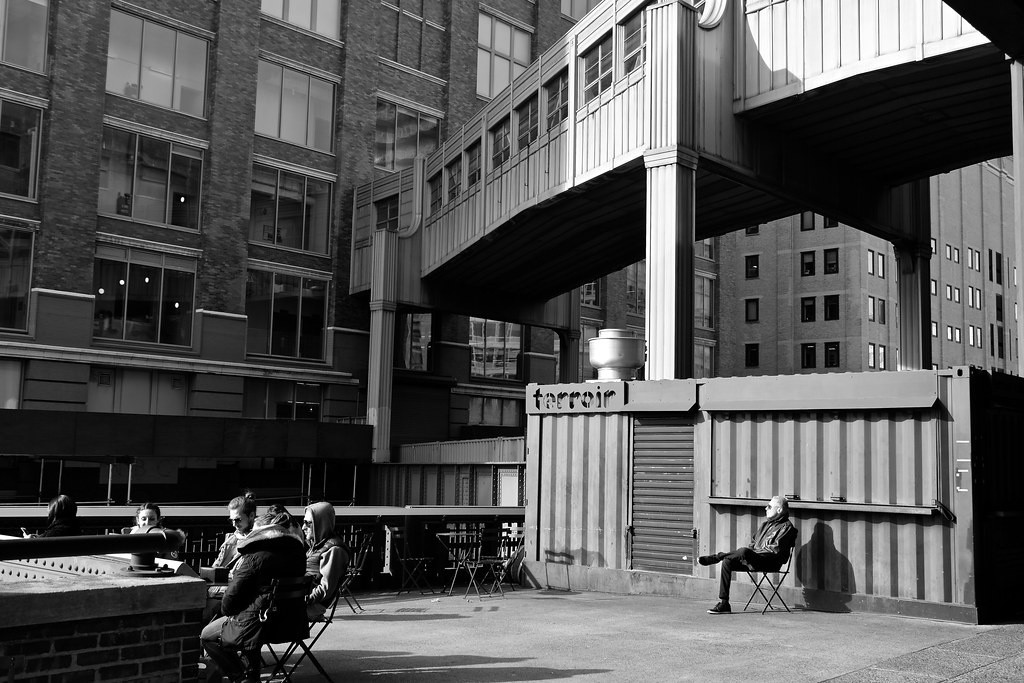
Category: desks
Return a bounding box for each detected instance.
[435,533,485,594]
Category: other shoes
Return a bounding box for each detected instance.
[697,551,724,566]
[707,601,731,613]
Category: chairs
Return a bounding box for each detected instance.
[247,529,375,683]
[743,528,798,615]
[388,529,529,598]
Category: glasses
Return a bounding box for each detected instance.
[228,518,242,524]
[766,504,780,509]
[303,520,313,527]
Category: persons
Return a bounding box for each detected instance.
[130,493,352,683]
[697,495,795,614]
[24,494,87,539]
